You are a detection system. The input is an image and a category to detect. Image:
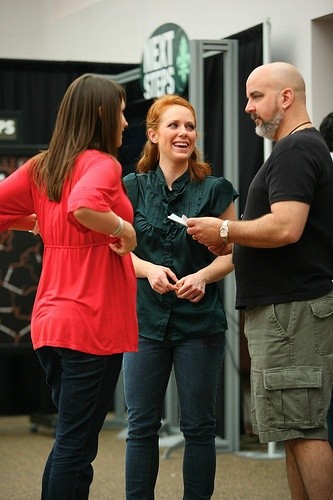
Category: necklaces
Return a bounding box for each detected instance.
[285,121,313,134]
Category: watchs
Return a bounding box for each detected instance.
[220,220,231,244]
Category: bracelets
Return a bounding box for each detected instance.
[107,216,124,238]
[28,213,41,235]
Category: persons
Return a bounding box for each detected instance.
[187,61,333,500]
[0,73,138,500]
[123,94,239,500]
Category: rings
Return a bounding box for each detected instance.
[192,235,197,240]
